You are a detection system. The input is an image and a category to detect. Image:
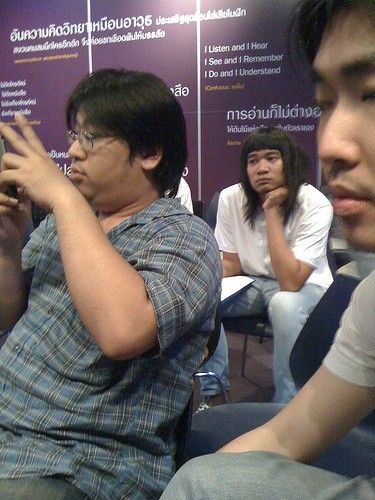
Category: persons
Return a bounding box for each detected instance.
[198,126,333,403]
[0,67,223,500]
[160,0,375,500]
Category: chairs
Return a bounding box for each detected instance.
[192,192,220,232]
[174,314,375,476]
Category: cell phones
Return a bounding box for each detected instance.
[0,133,17,199]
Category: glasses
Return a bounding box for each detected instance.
[67,129,113,150]
